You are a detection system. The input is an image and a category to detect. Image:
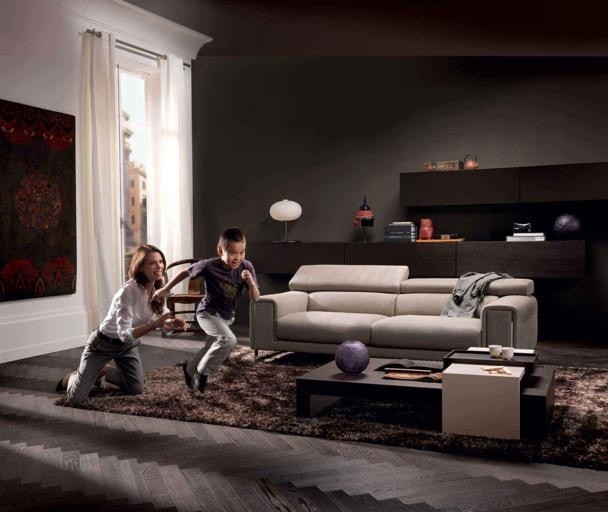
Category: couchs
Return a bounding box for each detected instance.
[249,263,540,363]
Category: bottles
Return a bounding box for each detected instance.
[354,194,374,227]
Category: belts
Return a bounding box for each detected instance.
[96,327,124,346]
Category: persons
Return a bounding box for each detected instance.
[148,226,262,398]
[53,243,187,405]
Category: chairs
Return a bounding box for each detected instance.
[161,256,206,330]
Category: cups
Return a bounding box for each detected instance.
[489,345,515,360]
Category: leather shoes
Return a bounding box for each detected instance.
[55,373,68,392]
[94,377,102,387]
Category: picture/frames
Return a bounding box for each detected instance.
[0,97,78,305]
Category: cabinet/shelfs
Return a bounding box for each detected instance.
[398,160,608,207]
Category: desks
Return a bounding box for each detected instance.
[268,239,598,346]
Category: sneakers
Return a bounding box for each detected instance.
[195,364,209,393]
[181,361,194,390]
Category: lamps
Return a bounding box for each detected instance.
[269,198,303,248]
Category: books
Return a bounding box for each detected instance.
[512,231,545,237]
[382,219,418,244]
[372,359,443,372]
[505,234,546,241]
[422,159,464,170]
[431,233,458,239]
[381,371,441,383]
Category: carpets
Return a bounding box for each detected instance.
[55,346,608,475]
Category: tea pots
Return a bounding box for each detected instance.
[464,153,479,170]
